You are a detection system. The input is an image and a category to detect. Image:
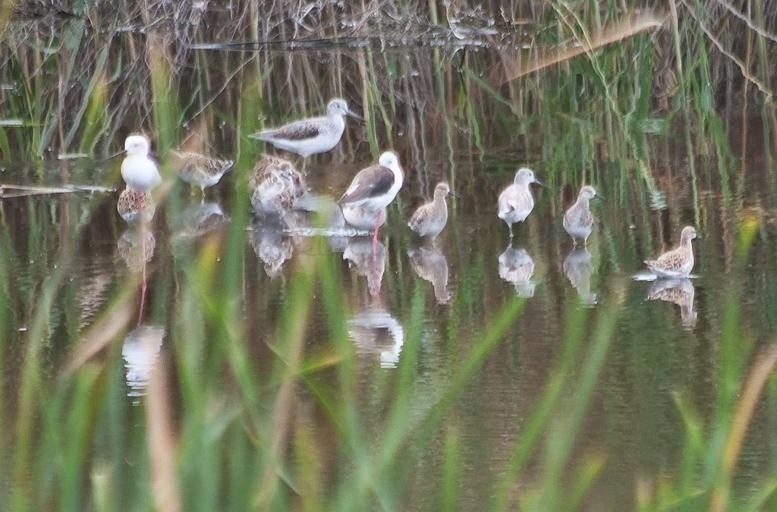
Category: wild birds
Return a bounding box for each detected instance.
[498,240,539,302]
[496,165,548,241]
[247,97,369,175]
[117,225,158,277]
[335,149,404,243]
[342,205,389,234]
[166,203,228,247]
[245,153,306,221]
[345,305,408,366]
[169,145,236,204]
[563,184,607,250]
[643,225,704,277]
[341,236,389,305]
[118,183,156,226]
[408,245,453,304]
[561,249,600,309]
[243,216,299,278]
[642,275,699,329]
[115,133,163,188]
[407,182,457,243]
